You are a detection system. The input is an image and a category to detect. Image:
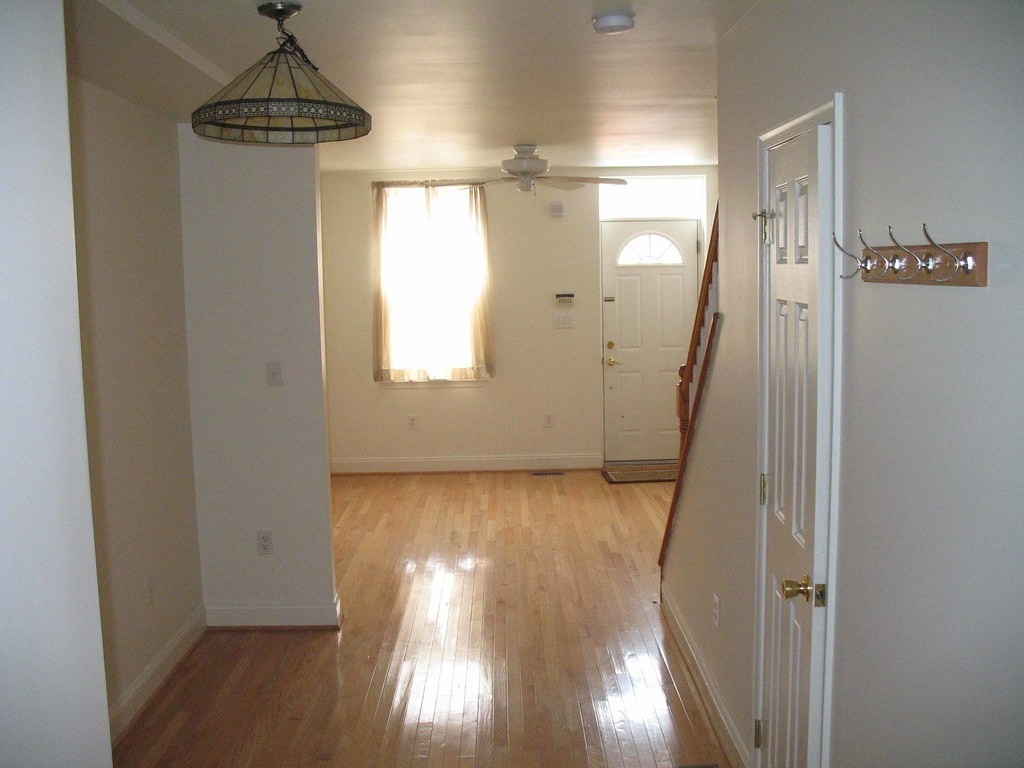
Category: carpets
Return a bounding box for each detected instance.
[600,468,678,484]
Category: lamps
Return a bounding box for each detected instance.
[192,2,372,146]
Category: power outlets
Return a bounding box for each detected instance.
[407,414,416,430]
[256,530,274,555]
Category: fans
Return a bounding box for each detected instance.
[457,143,627,194]
